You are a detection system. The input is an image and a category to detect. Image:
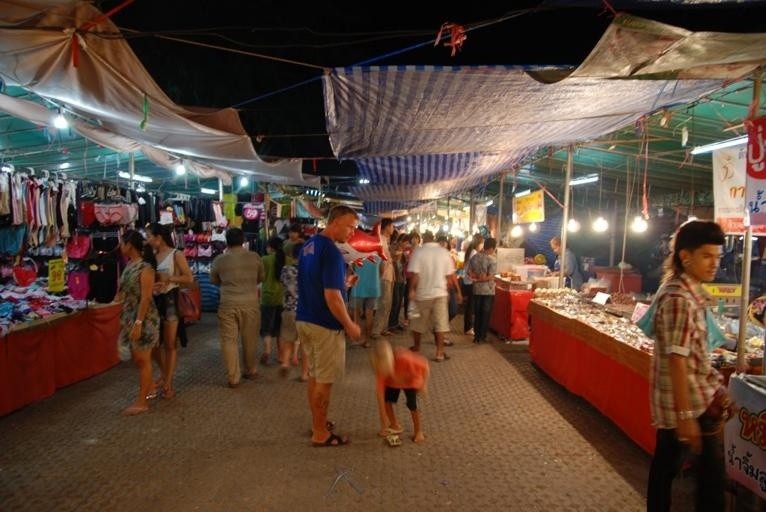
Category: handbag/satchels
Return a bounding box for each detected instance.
[178,278,201,320]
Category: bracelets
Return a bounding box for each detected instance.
[676,410,697,419]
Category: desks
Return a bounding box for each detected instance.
[0,301,124,418]
[491,286,578,341]
[527,293,766,471]
[593,266,643,293]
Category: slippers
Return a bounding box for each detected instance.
[121,406,148,416]
[378,427,402,447]
[312,420,348,445]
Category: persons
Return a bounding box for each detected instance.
[636,218,739,512]
[294,206,361,448]
[551,236,582,293]
[368,340,428,441]
[209,218,497,389]
[114,222,194,417]
